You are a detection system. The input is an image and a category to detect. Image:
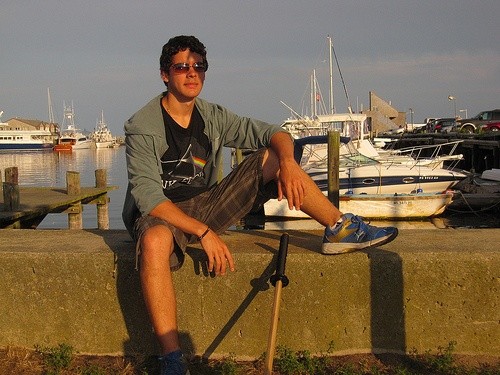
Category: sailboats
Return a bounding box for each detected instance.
[0,87,126,149]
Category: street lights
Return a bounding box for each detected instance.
[408,107,414,130]
[448,96,456,117]
[460,109,468,120]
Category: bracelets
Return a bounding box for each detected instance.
[197,225,212,241]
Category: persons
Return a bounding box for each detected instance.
[122,36,398,375]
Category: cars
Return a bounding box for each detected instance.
[434,109,500,134]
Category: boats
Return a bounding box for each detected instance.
[230,34,500,219]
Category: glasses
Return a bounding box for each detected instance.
[168,61,207,73]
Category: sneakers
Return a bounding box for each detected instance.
[160,350,190,375]
[322,212,399,256]
